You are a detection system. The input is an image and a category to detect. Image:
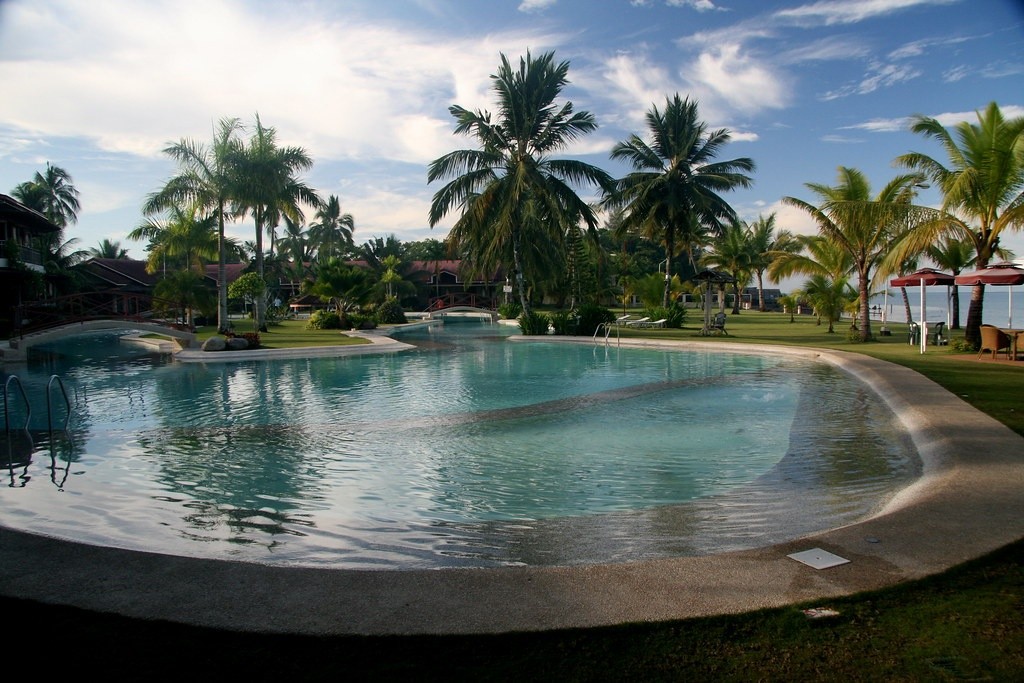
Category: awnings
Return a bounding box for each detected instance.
[890,268,954,289]
[955,261,1024,286]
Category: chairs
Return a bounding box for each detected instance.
[932,321,945,345]
[908,321,921,345]
[616,315,668,330]
[977,324,1011,361]
[712,313,728,336]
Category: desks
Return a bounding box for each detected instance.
[1000,328,1024,361]
